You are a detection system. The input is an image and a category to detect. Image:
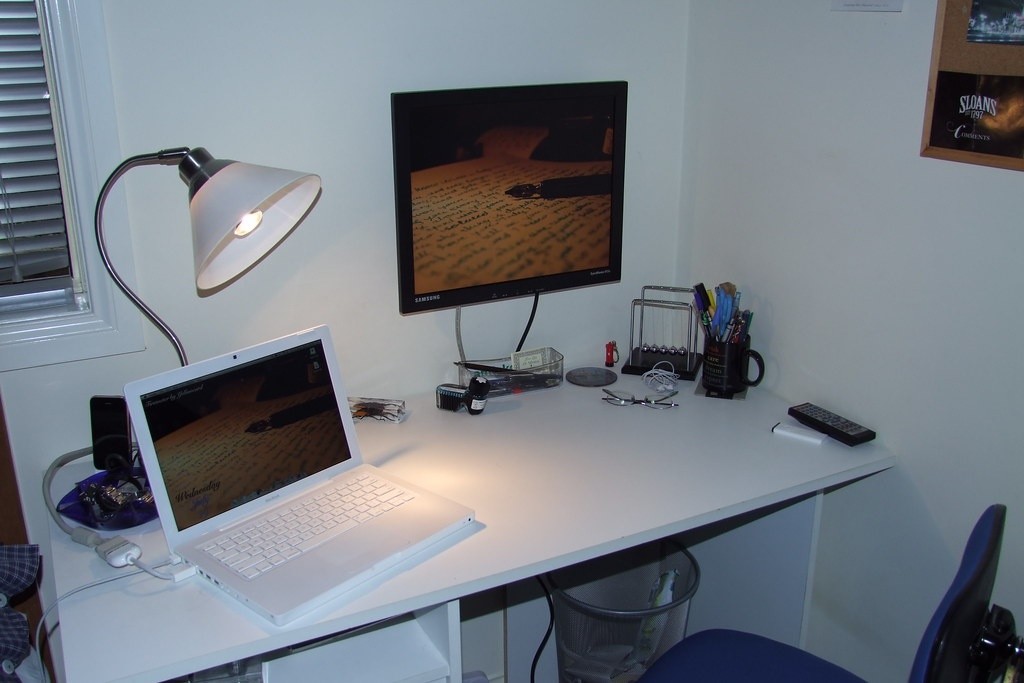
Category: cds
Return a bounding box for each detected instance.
[566,367,617,387]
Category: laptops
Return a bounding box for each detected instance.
[123,325,475,626]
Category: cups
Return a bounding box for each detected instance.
[701,333,765,394]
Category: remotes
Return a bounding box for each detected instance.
[772,422,827,445]
[788,402,876,447]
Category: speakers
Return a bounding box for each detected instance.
[90,395,134,471]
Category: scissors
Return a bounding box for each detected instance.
[719,287,733,337]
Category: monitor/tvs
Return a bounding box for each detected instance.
[390,80,629,316]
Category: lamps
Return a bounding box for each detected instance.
[96,147,322,368]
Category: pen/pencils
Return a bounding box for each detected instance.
[243,391,337,433]
[504,172,612,198]
[694,279,754,343]
[452,361,562,397]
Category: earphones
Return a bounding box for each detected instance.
[665,384,674,391]
[657,385,665,392]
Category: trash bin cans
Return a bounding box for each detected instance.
[548,537,702,683]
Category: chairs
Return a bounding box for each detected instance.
[632,504,1024,683]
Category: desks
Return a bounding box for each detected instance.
[49,358,897,683]
